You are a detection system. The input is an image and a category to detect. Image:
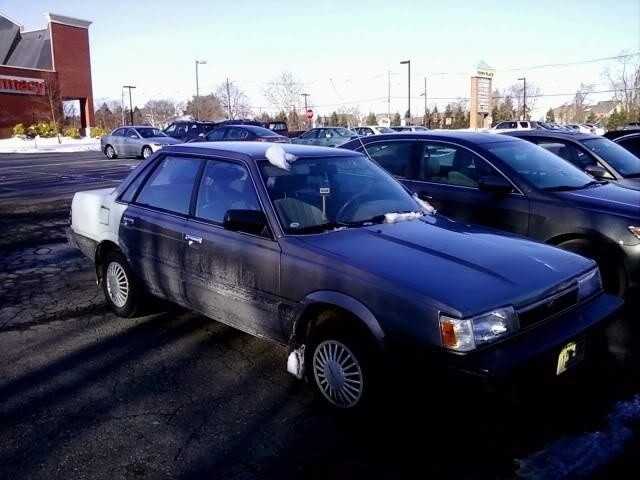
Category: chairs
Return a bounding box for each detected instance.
[448,151,477,187]
[266,173,327,229]
[425,155,447,184]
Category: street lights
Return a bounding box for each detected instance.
[300,93,308,119]
[420,92,427,113]
[516,77,526,120]
[400,59,411,123]
[123,85,137,125]
[193,58,207,122]
